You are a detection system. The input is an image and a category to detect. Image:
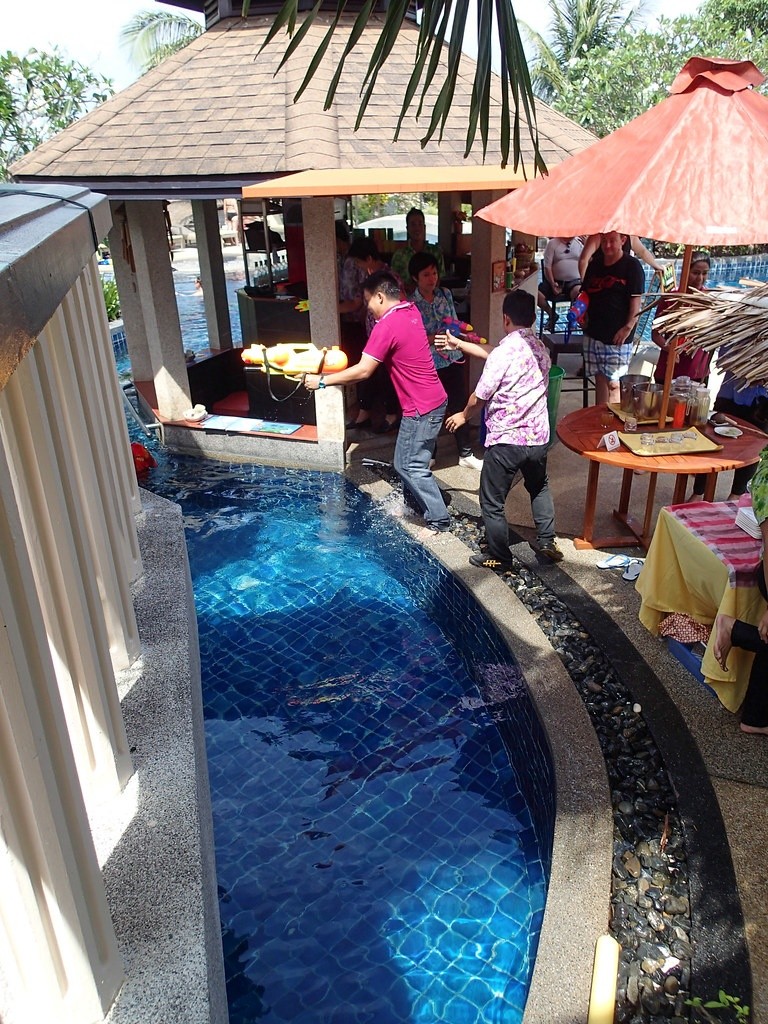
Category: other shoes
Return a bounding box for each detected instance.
[545,312,560,330]
[577,367,584,376]
[374,418,400,434]
[346,416,372,430]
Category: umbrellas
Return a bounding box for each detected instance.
[473,56,768,536]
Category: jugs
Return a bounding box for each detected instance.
[686,388,711,426]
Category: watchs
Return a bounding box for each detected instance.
[319,377,325,388]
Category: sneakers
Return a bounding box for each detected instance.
[469,551,507,570]
[540,542,564,562]
[430,457,435,469]
[459,454,483,471]
[425,520,450,532]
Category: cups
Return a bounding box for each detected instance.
[601,412,614,428]
[354,228,386,239]
[625,414,638,432]
[640,432,698,454]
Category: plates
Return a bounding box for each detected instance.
[707,411,729,427]
[735,506,763,539]
[714,426,743,436]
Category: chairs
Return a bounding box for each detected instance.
[538,259,576,338]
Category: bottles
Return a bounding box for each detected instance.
[388,228,394,240]
[672,375,692,429]
[506,240,516,289]
[253,255,288,288]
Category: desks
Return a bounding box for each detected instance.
[635,493,765,712]
[555,394,768,556]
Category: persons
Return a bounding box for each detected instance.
[577,232,664,377]
[579,231,646,403]
[537,236,584,330]
[407,252,473,467]
[714,444,768,734]
[335,220,370,343]
[686,346,768,502]
[304,272,450,532]
[446,289,565,572]
[651,251,717,388]
[345,236,406,433]
[391,208,447,293]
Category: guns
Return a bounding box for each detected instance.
[294,300,310,313]
[566,291,590,328]
[437,316,487,345]
[240,342,348,383]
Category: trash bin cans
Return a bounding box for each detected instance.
[548,365,565,442]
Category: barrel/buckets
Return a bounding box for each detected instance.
[547,362,566,445]
[631,382,664,422]
[619,374,651,413]
